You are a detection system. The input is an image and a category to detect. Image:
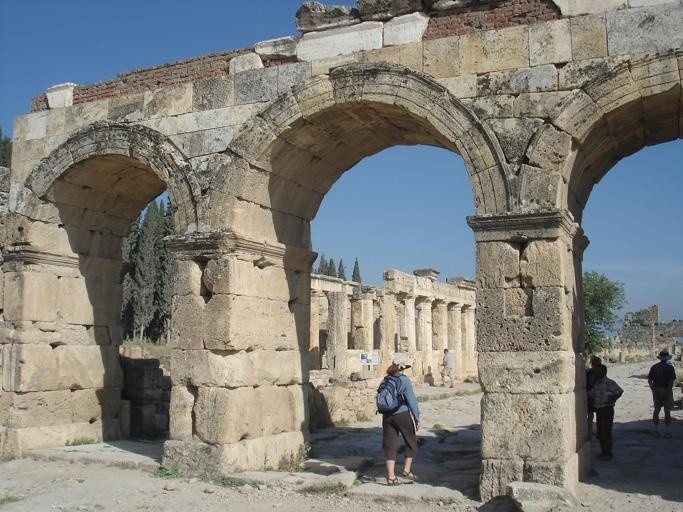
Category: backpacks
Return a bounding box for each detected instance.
[376,373,407,415]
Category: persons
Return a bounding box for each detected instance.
[586,364,625,461]
[646,348,678,437]
[440,348,454,388]
[375,355,420,485]
[586,353,601,438]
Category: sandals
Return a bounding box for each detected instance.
[388,477,405,486]
[402,471,419,480]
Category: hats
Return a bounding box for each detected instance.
[392,356,411,369]
[656,350,672,361]
